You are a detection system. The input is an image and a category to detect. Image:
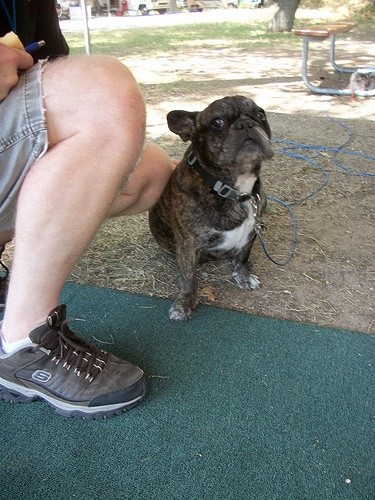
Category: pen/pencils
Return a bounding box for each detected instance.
[23,40,45,52]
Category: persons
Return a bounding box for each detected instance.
[0,0,179,419]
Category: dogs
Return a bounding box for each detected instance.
[148,95,275,322]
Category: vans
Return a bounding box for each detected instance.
[126,0,171,16]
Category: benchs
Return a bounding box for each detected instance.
[290,21,375,97]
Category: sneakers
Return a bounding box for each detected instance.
[1,303,148,420]
